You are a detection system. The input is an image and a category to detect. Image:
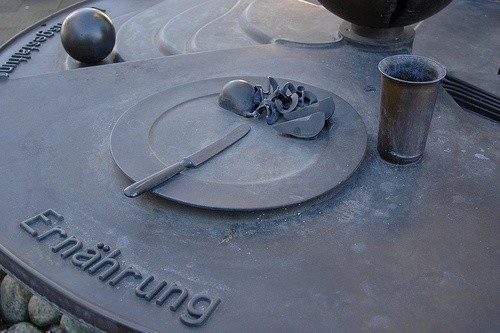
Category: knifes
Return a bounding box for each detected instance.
[123,124,251,198]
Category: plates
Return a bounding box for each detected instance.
[110,75,368,210]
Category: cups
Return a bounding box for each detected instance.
[377,55,447,163]
